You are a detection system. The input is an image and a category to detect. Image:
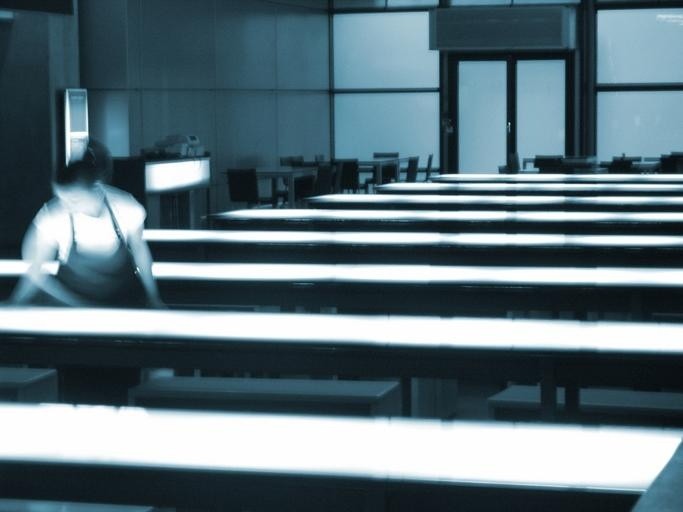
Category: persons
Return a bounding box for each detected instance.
[6,161,165,309]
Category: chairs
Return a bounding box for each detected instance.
[226,154,433,207]
[497,152,683,173]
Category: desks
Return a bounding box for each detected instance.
[0,173,683,512]
[112,153,214,229]
